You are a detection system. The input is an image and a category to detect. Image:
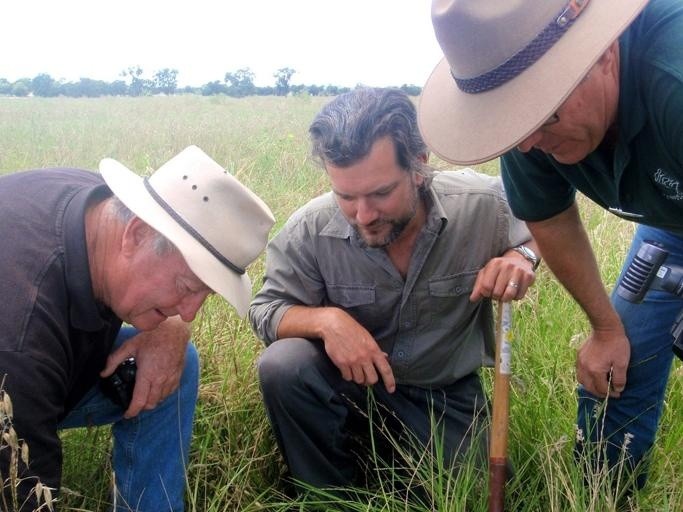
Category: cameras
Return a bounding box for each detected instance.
[96,354,136,410]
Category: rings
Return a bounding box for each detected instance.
[508,281,519,288]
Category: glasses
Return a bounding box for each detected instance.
[102,360,137,409]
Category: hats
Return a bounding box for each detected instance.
[415,0,648,167]
[98,145,276,319]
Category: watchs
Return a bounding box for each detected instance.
[511,245,536,270]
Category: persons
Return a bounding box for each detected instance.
[0,144,279,512]
[245,77,544,507]
[417,1,680,512]
